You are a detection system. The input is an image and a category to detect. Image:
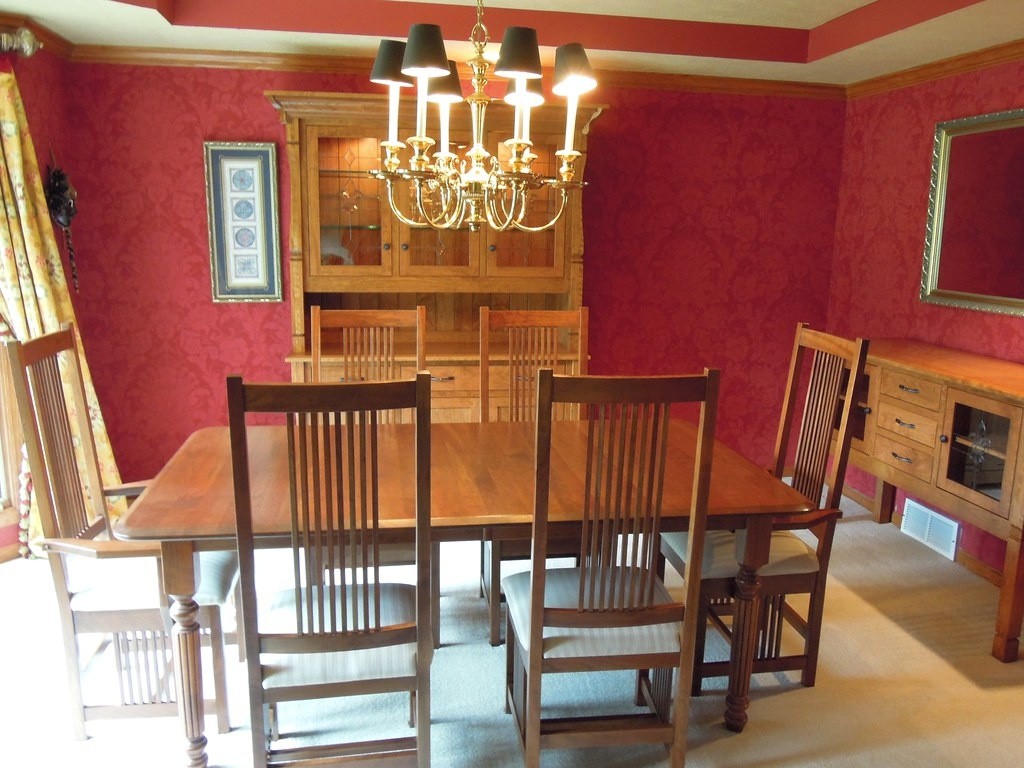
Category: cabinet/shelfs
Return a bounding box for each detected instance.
[264,91,609,427]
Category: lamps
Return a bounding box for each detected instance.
[370,0,596,233]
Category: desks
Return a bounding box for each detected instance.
[112,416,817,768]
[829,338,1024,661]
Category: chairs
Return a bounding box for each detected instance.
[308,304,441,649]
[6,321,248,740]
[649,322,869,696]
[501,366,719,768]
[480,305,611,646]
[226,371,434,768]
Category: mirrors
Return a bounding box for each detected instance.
[919,109,1024,319]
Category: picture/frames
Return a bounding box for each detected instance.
[202,140,284,303]
